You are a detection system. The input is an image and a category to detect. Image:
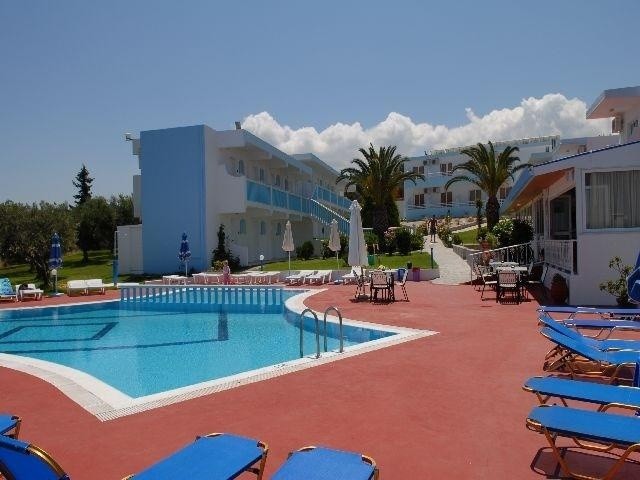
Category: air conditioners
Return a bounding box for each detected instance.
[612,116,622,133]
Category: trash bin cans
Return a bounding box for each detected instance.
[407,263,412,269]
[413,267,420,282]
[398,268,405,281]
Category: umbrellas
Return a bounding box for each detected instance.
[328,219,341,282]
[282,220,294,276]
[179,232,191,277]
[48,233,63,293]
[348,200,369,295]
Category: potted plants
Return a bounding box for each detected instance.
[550,274,570,305]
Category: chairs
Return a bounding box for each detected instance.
[522,305,640,480]
[286,269,332,286]
[342,266,365,286]
[66,278,106,296]
[162,270,280,286]
[352,268,409,306]
[1,410,23,438]
[269,443,380,480]
[0,278,44,301]
[470,255,549,306]
[2,430,271,479]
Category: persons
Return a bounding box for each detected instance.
[19,283,34,290]
[222,260,230,285]
[428,214,437,243]
